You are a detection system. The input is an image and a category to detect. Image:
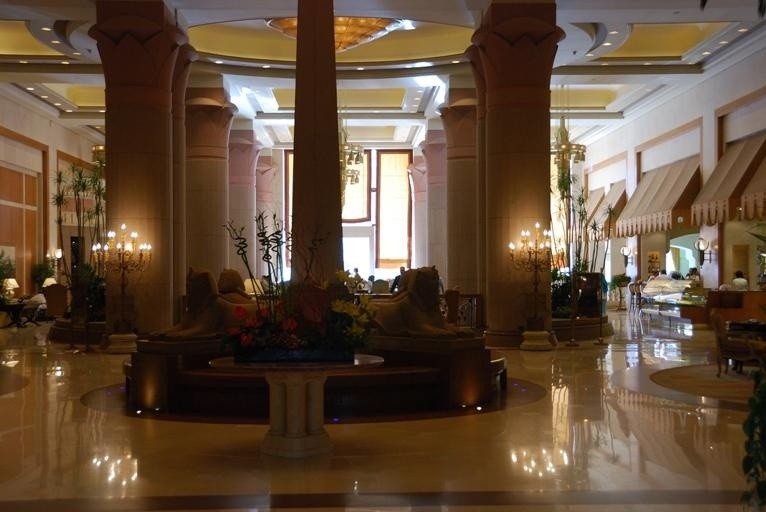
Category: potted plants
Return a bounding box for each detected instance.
[754,245,766,290]
[46,147,104,346]
[609,273,630,290]
[551,167,615,343]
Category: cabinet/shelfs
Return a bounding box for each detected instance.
[640,286,711,324]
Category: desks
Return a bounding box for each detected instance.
[725,320,766,373]
[209,353,387,461]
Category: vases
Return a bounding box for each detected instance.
[234,329,355,363]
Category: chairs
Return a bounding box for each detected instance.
[629,282,645,310]
[0,277,70,331]
[709,309,766,377]
[186,265,444,336]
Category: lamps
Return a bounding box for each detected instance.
[263,15,403,56]
[549,81,587,165]
[339,105,366,210]
[620,246,633,266]
[92,225,153,354]
[508,222,560,350]
[693,238,712,267]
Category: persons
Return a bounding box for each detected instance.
[354,268,359,277]
[368,276,374,287]
[390,267,406,293]
[731,271,749,292]
[648,267,700,283]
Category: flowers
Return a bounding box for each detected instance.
[222,209,330,345]
